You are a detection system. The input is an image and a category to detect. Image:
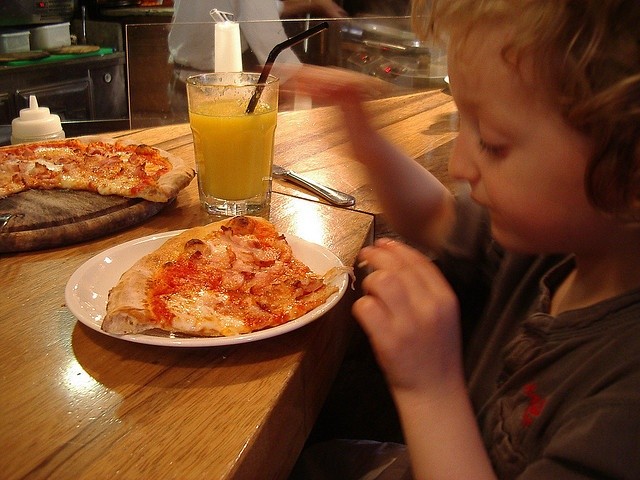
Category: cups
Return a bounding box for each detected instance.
[187,73,280,223]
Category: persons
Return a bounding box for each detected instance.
[168,0,389,122]
[338,0,640,480]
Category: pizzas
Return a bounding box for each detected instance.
[1,139,195,205]
[100,211,341,336]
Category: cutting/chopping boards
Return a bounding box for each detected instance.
[0,188,174,254]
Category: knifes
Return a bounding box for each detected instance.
[273,164,355,208]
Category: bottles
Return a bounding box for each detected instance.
[10,96,66,144]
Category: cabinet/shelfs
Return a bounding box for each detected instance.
[1,50,125,137]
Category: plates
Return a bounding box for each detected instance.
[65,227,349,347]
